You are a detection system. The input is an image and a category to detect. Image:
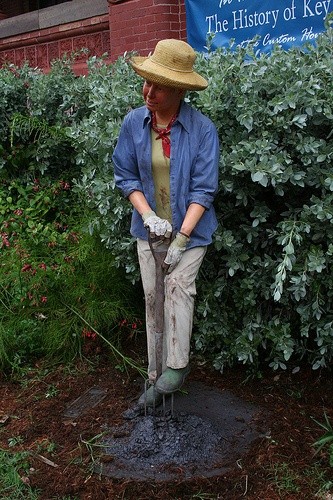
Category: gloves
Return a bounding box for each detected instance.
[163,232,189,274]
[142,211,173,238]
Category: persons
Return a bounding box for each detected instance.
[110,64,218,407]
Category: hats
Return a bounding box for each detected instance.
[129,39,208,91]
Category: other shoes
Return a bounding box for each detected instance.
[138,383,167,408]
[156,363,191,393]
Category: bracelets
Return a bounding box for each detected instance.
[178,232,189,238]
